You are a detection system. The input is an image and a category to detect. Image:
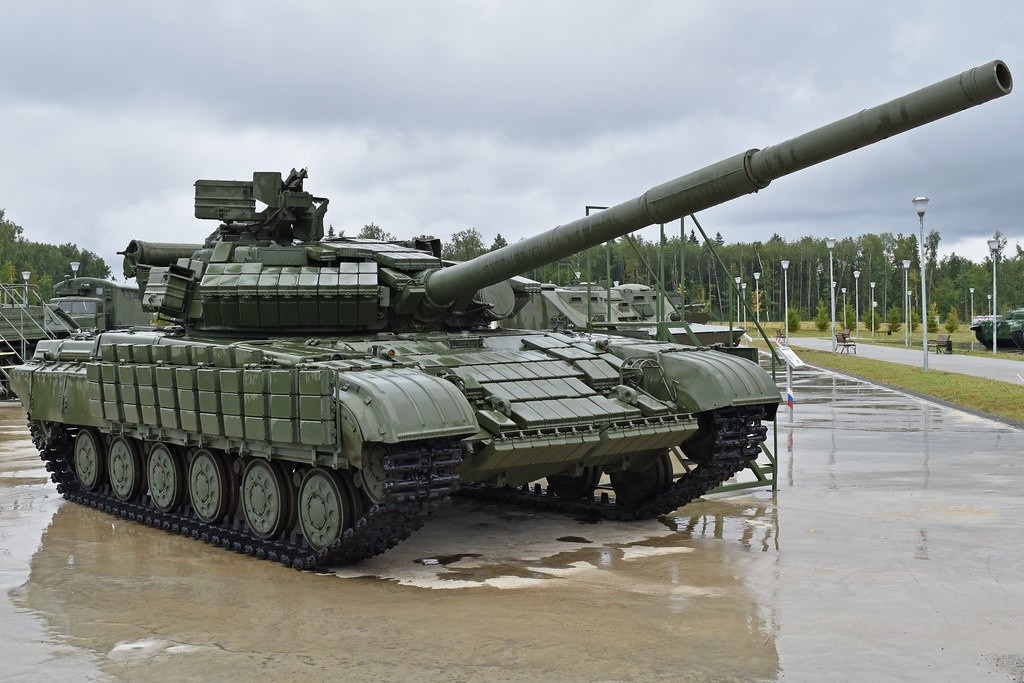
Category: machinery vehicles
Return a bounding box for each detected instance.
[971,309,1024,349]
[7,58,1012,552]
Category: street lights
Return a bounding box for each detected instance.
[901,259,911,344]
[969,288,973,350]
[752,272,762,339]
[986,238,999,353]
[824,238,838,353]
[734,276,740,329]
[741,283,747,331]
[21,271,31,304]
[852,269,861,340]
[870,281,876,337]
[908,290,913,336]
[780,259,791,347]
[987,295,991,315]
[70,261,79,278]
[841,287,848,330]
[911,196,929,372]
[574,271,580,279]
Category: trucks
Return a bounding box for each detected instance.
[43,276,153,338]
[1,283,45,383]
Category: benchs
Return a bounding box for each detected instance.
[922,334,951,354]
[839,328,851,342]
[775,329,786,343]
[835,334,856,354]
